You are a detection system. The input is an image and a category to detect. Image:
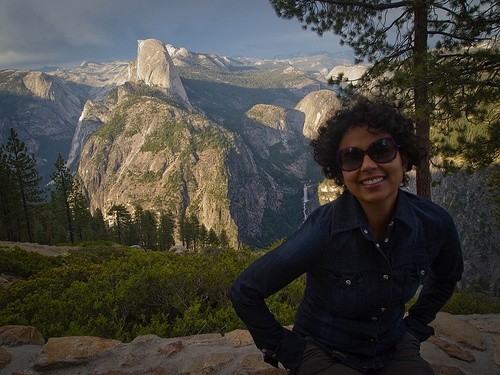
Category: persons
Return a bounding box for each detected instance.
[229,100,465,375]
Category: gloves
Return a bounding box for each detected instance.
[249,321,309,371]
[398,309,434,341]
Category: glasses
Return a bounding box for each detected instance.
[332,135,398,172]
